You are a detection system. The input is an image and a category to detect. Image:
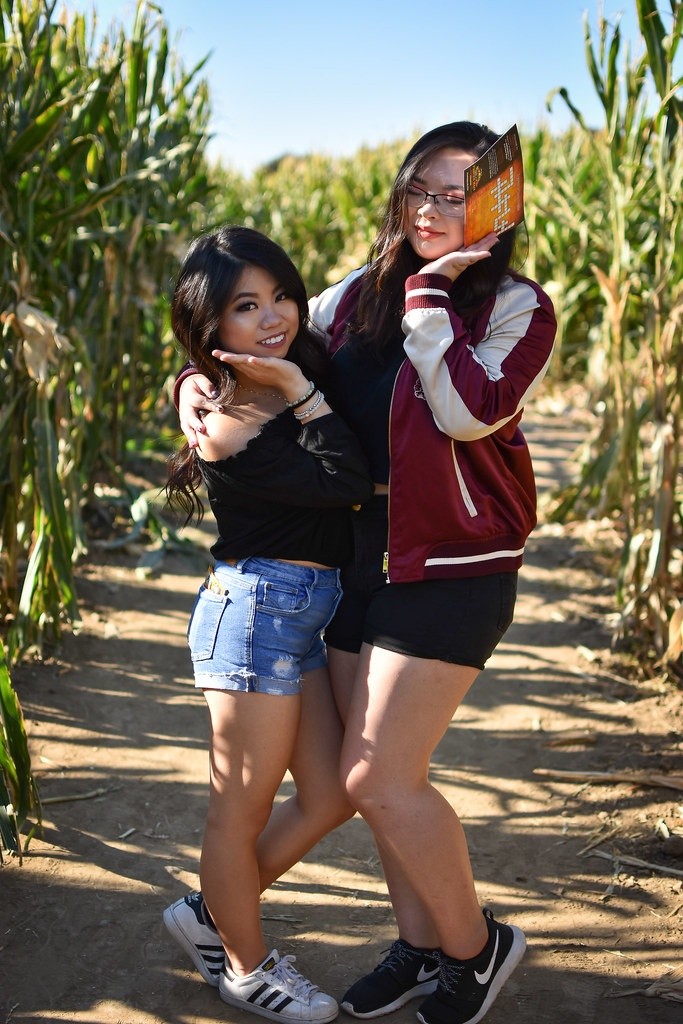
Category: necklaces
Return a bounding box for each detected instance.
[238,385,289,402]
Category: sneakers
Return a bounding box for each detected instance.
[219,949,339,1023]
[339,940,441,1019]
[162,891,228,987]
[416,907,526,1023]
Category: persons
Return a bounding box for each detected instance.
[173,121,558,1024]
[162,226,376,1024]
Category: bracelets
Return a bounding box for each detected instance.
[285,381,324,420]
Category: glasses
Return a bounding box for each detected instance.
[406,184,464,218]
